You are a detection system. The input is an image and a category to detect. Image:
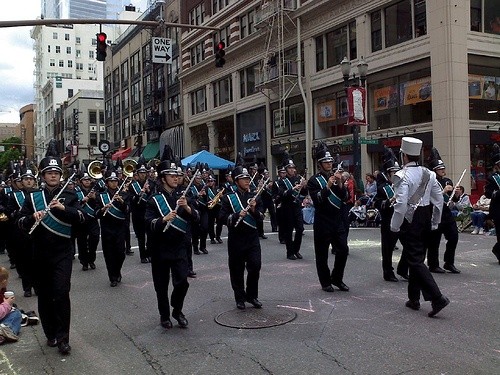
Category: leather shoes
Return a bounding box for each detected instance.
[286,251,303,260]
[428,294,450,317]
[428,265,445,273]
[385,277,398,282]
[160,316,172,329]
[247,296,263,306]
[322,284,334,292]
[57,342,71,353]
[172,309,188,326]
[397,271,409,279]
[235,301,245,310]
[4,224,289,298]
[443,262,460,273]
[336,282,349,291]
[46,335,56,348]
[405,300,420,309]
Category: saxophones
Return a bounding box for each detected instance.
[207,187,225,209]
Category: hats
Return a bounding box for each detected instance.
[381,144,401,171]
[316,138,334,163]
[428,147,446,171]
[399,137,422,156]
[5,138,297,191]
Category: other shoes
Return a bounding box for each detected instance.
[393,245,399,250]
[0,324,17,339]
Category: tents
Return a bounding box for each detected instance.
[180,150,235,169]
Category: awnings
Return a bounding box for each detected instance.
[62,154,70,165]
[112,147,132,161]
[142,142,160,161]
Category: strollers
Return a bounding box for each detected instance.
[349,205,367,227]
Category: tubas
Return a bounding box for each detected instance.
[87,160,105,180]
[121,159,139,177]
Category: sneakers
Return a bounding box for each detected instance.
[479,227,484,235]
[471,228,479,234]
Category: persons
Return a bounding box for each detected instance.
[484,146,500,265]
[64,153,160,287]
[0,139,89,352]
[144,144,224,327]
[451,186,473,232]
[342,170,361,226]
[377,137,461,317]
[471,195,491,235]
[216,161,273,309]
[269,148,308,260]
[308,139,350,292]
[0,264,22,344]
[302,195,315,224]
[349,170,381,228]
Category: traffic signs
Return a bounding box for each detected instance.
[151,37,172,64]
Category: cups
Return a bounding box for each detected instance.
[4,291,14,299]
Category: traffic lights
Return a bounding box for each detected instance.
[215,42,225,67]
[96,33,107,61]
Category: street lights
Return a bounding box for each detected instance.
[340,56,368,199]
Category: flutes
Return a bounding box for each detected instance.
[27,171,76,235]
[102,175,129,217]
[249,171,258,186]
[389,198,396,208]
[291,168,307,203]
[446,168,466,207]
[333,161,343,175]
[162,168,200,232]
[234,176,270,227]
[137,179,149,204]
[80,183,97,209]
